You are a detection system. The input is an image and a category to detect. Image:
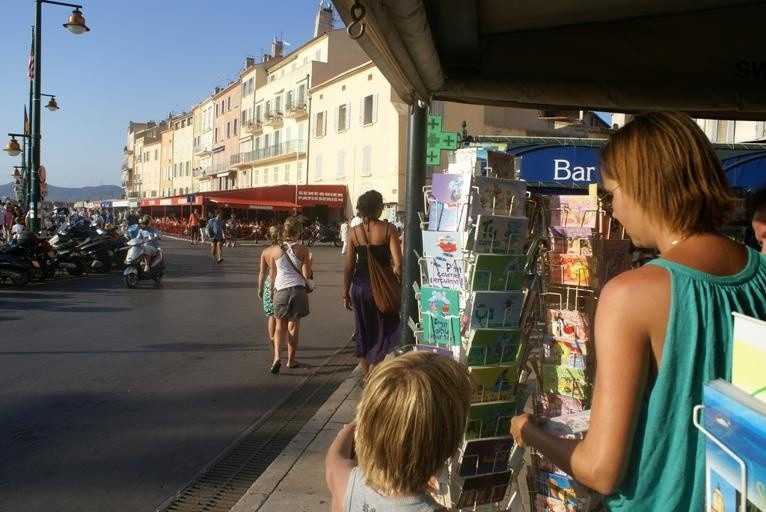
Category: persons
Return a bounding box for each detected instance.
[511,111,766,511]
[269,216,316,372]
[188,208,240,263]
[748,205,766,253]
[323,351,478,512]
[314,216,321,230]
[135,215,159,272]
[252,220,261,245]
[342,190,401,387]
[256,225,287,346]
[0,198,141,238]
[339,212,404,254]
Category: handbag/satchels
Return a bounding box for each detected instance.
[357,222,401,315]
[305,278,314,294]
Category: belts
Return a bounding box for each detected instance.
[281,285,304,291]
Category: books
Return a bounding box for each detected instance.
[702,376,766,512]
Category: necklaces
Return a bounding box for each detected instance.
[664,235,686,254]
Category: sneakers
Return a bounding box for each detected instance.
[270,358,281,374]
[143,263,153,274]
[286,361,302,369]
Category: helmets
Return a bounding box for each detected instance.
[138,214,153,231]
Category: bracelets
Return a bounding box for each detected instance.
[343,295,350,299]
[270,288,273,291]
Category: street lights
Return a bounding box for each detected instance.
[25,0,89,225]
[6,91,62,209]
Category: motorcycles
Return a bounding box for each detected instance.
[121,232,166,289]
[307,216,343,248]
[0,207,127,288]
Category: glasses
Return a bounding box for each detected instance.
[596,183,621,209]
